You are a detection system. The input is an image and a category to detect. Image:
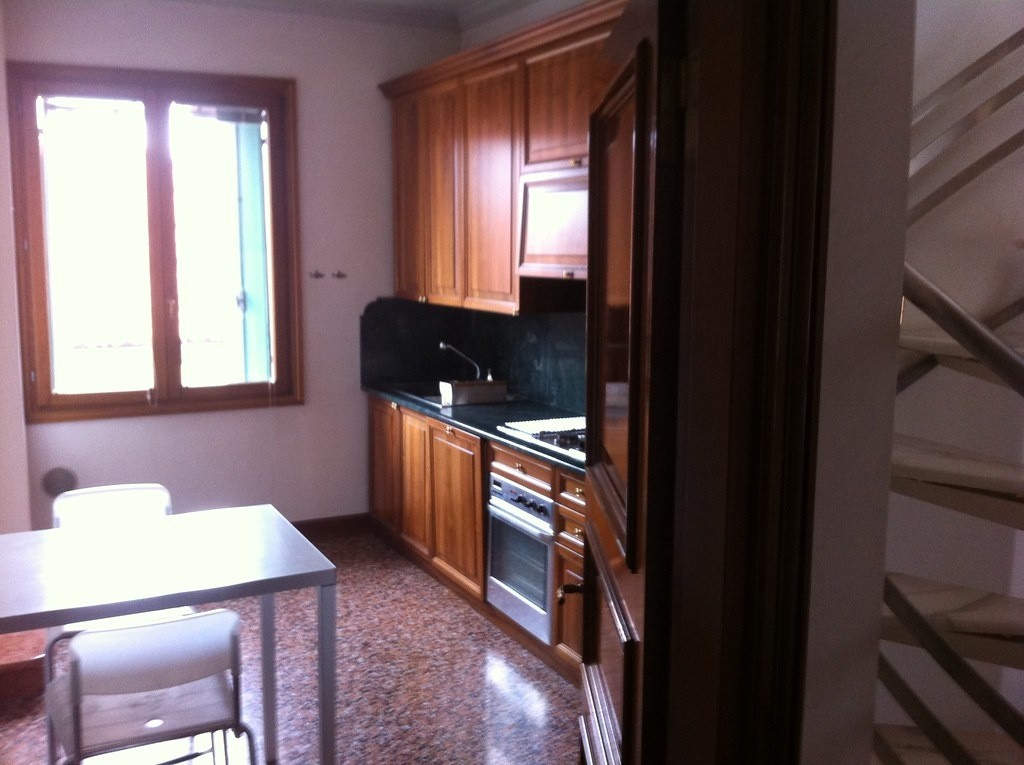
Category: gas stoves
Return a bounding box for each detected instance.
[497,415,587,465]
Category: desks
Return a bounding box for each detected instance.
[0,502,342,765]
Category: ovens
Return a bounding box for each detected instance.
[482,472,561,647]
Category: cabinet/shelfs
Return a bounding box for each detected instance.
[369,396,595,692]
[377,1,626,319]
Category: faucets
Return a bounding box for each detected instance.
[439,341,482,380]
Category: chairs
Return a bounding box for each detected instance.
[39,483,258,765]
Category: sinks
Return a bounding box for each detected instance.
[393,387,464,408]
